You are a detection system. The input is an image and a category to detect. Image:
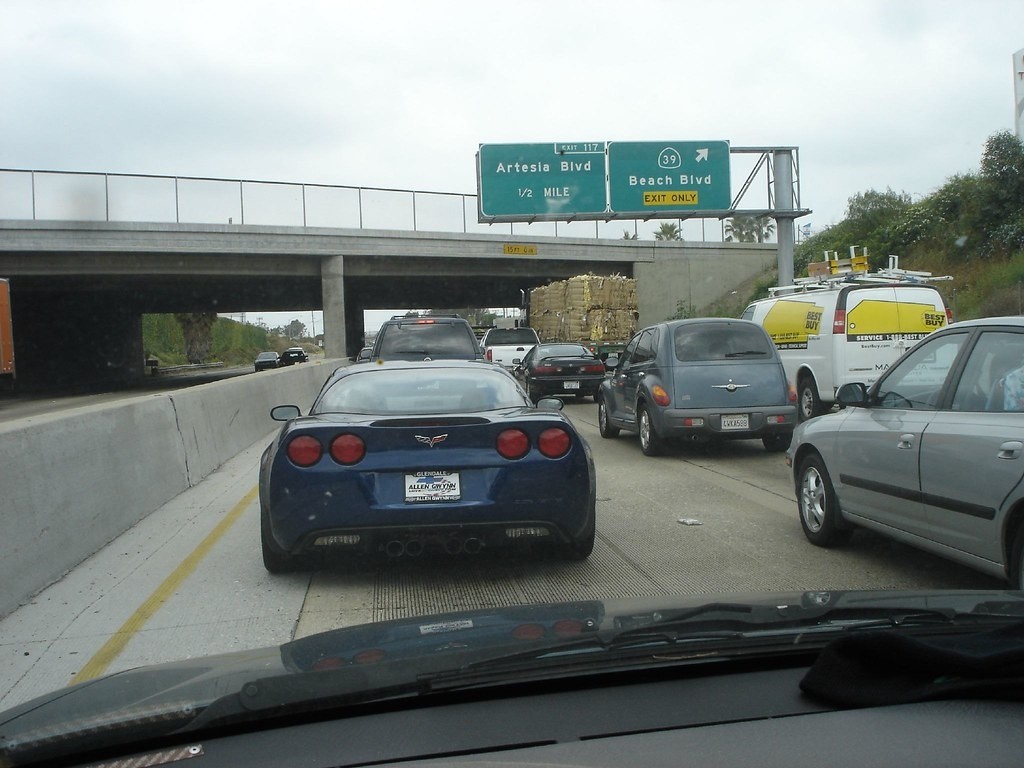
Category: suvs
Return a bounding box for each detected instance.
[360,315,486,361]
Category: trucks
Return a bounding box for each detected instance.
[520,286,638,367]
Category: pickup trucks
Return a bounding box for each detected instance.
[479,327,541,371]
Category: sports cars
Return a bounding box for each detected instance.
[258,356,597,574]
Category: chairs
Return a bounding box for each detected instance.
[364,376,479,410]
[985,343,1024,411]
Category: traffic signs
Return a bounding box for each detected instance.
[478,140,733,215]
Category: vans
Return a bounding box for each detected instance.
[740,278,955,421]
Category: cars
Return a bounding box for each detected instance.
[782,316,1024,587]
[598,317,799,455]
[254,351,280,371]
[279,349,309,365]
[512,344,607,403]
